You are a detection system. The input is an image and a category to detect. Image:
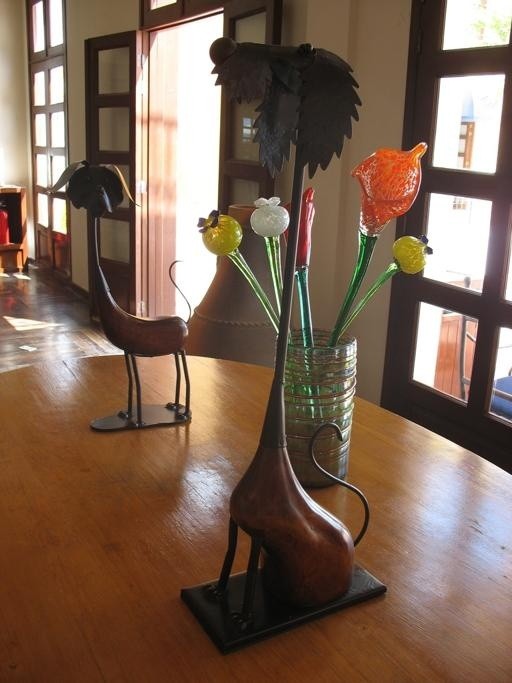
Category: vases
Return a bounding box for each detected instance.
[273,327,358,488]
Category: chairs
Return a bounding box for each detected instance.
[455,272,512,414]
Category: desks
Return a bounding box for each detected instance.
[0,355,512,682]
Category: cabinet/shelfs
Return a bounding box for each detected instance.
[0,186,30,275]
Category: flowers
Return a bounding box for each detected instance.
[194,142,433,413]
